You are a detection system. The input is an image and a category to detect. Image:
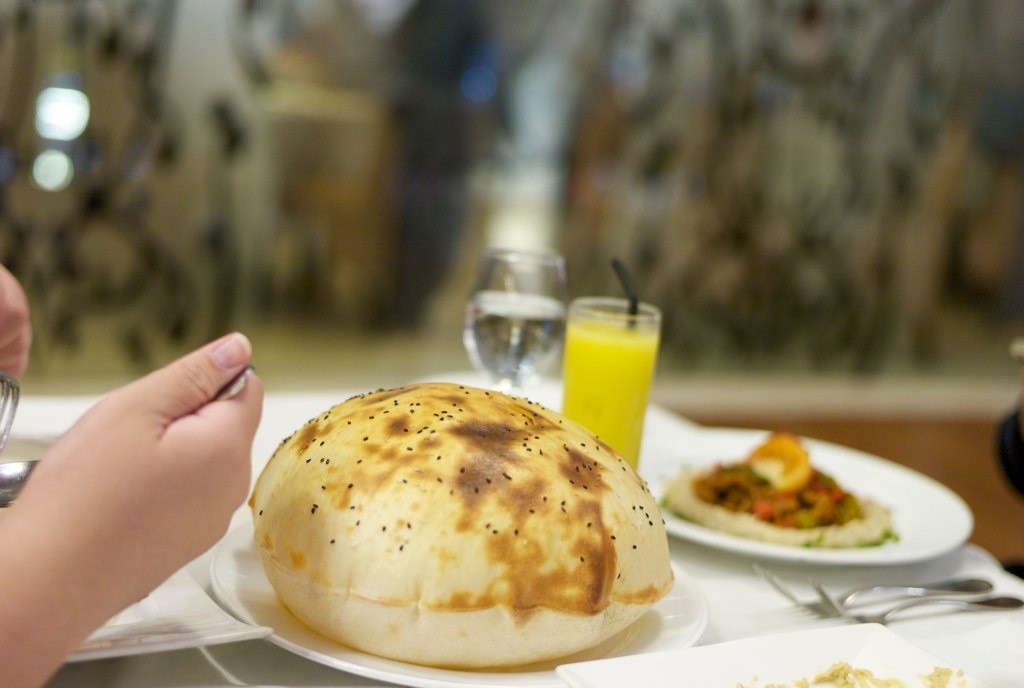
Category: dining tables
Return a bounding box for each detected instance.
[2,366,1024,688]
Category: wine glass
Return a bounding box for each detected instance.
[463,247,566,397]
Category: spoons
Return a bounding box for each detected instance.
[810,577,1022,623]
[0,376,255,509]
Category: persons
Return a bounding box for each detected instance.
[1,261,266,688]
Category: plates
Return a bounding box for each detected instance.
[639,421,974,566]
[209,524,707,688]
[55,562,270,665]
[554,623,986,688]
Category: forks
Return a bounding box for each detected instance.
[1,370,20,448]
[750,558,993,616]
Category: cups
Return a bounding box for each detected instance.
[562,295,662,476]
